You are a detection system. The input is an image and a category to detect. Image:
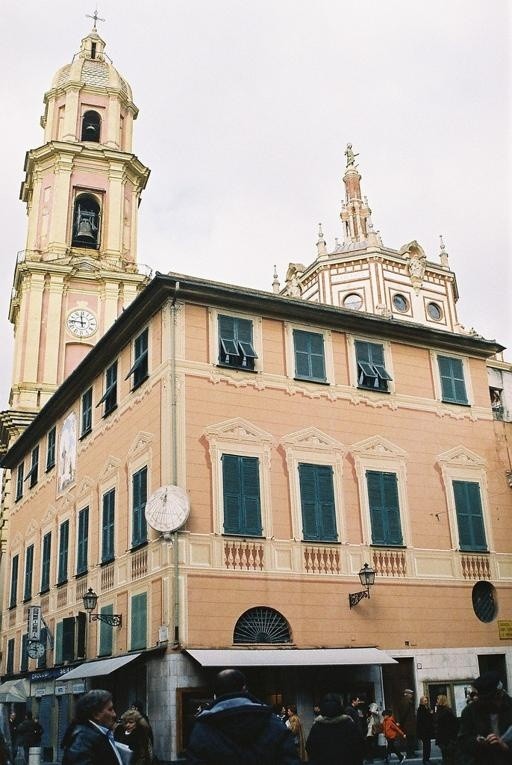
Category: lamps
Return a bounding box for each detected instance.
[349,562,377,608]
[82,586,122,629]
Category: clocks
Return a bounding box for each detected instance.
[65,307,99,340]
[27,641,46,659]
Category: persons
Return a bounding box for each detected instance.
[9,711,21,761]
[34,715,45,748]
[59,687,154,765]
[188,668,511,765]
[15,711,37,765]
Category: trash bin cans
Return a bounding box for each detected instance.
[29,747,42,765]
